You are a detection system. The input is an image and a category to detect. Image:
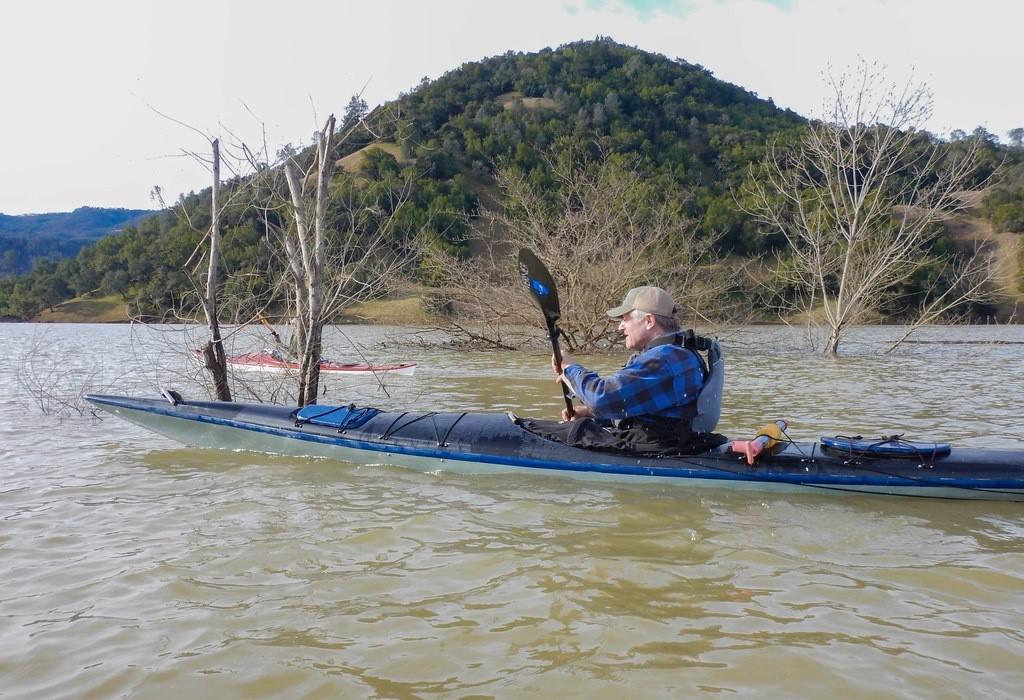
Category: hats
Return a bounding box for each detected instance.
[606,287,674,318]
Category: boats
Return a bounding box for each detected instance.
[84,391,1024,505]
[189,346,418,376]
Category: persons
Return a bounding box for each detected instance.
[269,318,298,363]
[551,286,707,451]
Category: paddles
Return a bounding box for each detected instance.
[517,246,576,419]
[255,312,282,344]
